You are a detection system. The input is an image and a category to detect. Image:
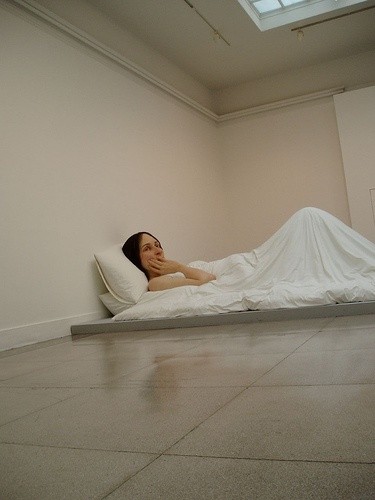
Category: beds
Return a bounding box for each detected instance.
[71,207,375,334]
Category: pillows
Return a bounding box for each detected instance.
[95,246,148,305]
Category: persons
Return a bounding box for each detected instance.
[122,206,352,292]
